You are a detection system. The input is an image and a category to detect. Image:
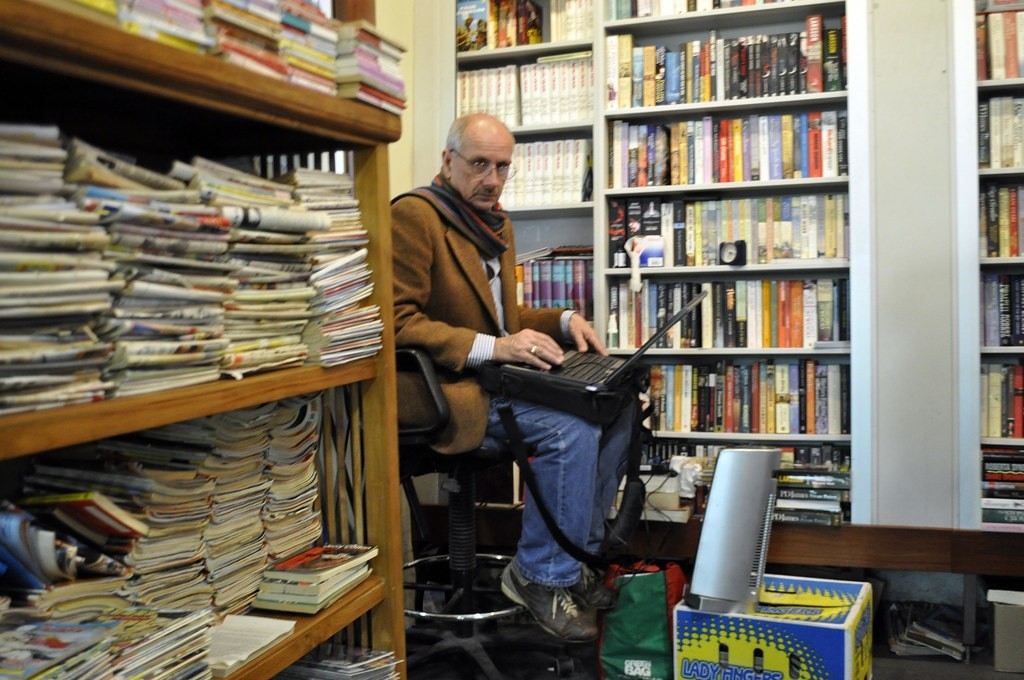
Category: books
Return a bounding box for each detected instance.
[0,0,408,680]
[456,0,1024,529]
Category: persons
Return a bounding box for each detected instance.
[390,115,637,641]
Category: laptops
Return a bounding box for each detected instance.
[497,291,708,392]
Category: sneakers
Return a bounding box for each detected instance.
[564,562,618,610]
[500,557,600,643]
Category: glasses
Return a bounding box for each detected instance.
[450,147,518,180]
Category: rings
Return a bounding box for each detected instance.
[530,346,537,353]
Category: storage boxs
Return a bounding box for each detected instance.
[991,601,1024,673]
[673,573,873,680]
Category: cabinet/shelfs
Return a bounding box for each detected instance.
[956,0,1024,533]
[455,0,598,330]
[0,0,407,680]
[598,0,880,525]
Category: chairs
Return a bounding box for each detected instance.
[391,347,583,680]
